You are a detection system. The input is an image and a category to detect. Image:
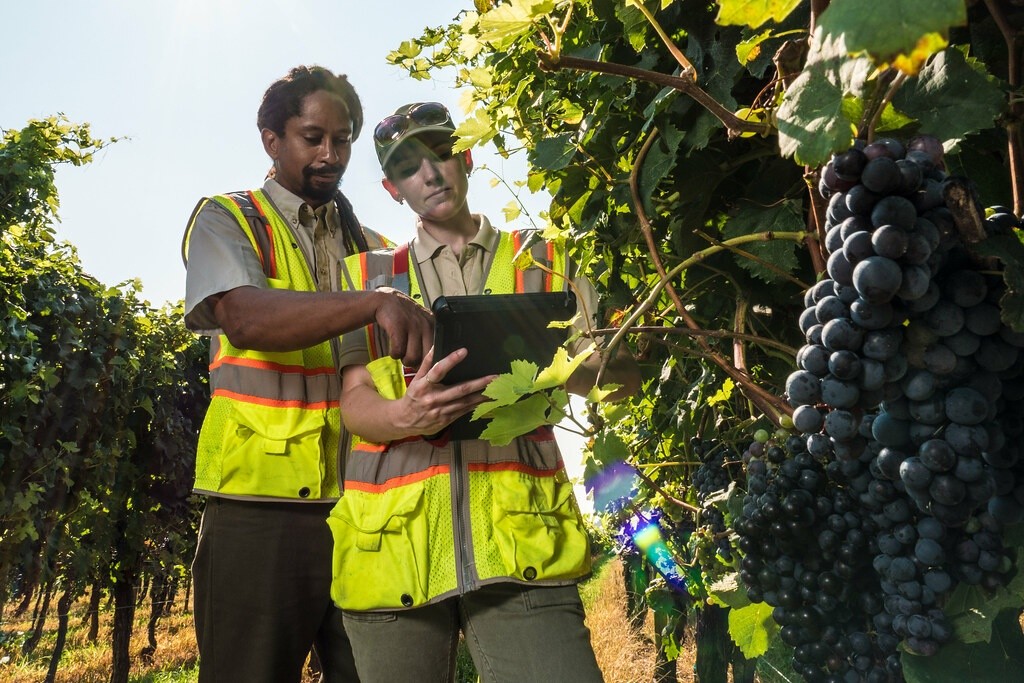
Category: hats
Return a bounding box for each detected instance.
[375,102,456,170]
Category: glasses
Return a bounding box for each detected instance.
[373,101,451,147]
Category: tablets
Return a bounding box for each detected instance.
[427,290,577,429]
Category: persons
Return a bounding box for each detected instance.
[326,101,644,683]
[181,64,434,683]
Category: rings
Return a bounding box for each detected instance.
[425,374,436,384]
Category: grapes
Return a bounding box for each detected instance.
[681,133,1024,683]
